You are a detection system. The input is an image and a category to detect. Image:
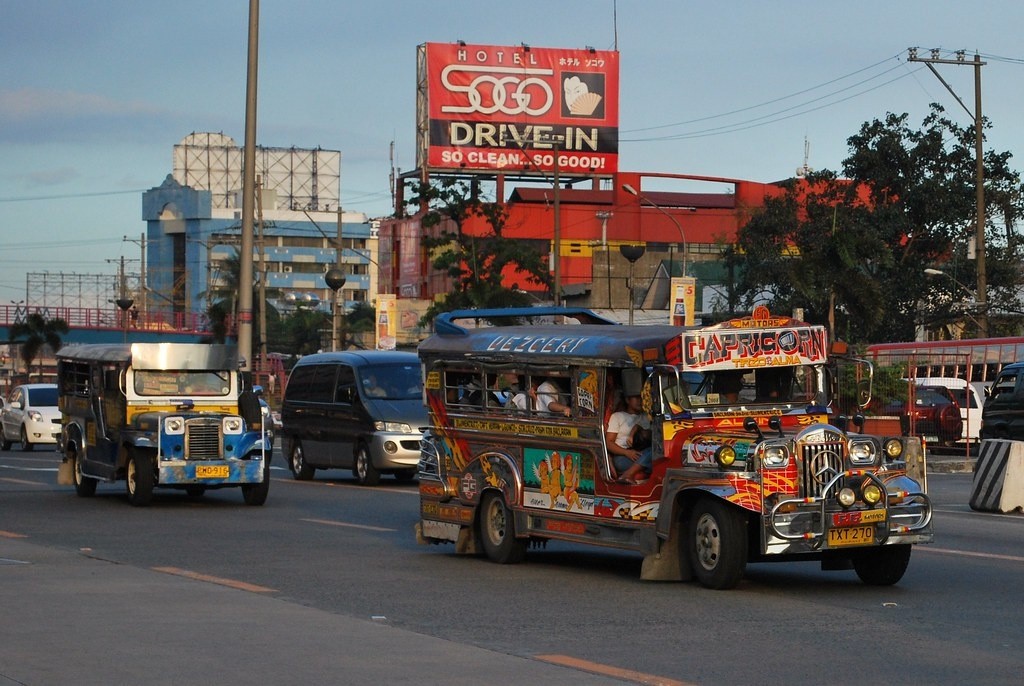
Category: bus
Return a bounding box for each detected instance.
[56,342,272,506]
[417,306,933,590]
[852,337,1024,405]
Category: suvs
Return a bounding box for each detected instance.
[839,378,963,448]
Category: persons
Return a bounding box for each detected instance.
[605,392,652,486]
[362,374,387,397]
[753,379,790,404]
[444,371,573,418]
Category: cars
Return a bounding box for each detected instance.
[0,396,6,447]
[3,383,63,451]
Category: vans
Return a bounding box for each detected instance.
[903,376,982,443]
[979,363,1024,441]
[280,350,428,485]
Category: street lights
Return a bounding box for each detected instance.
[925,269,988,339]
[11,299,23,325]
[621,184,686,278]
[330,237,389,294]
